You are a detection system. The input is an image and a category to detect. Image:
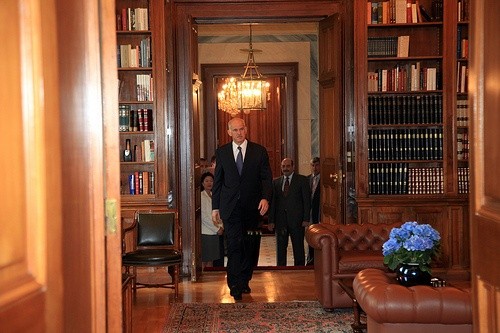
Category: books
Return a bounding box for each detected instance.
[116,6,155,195]
[367,0,470,195]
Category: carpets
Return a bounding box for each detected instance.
[163,300,368,333]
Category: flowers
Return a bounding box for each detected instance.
[382,221,442,276]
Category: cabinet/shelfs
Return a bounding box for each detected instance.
[364,0,470,198]
[117,30,157,199]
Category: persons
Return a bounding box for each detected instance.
[306,156,321,266]
[211,117,274,302]
[267,158,310,266]
[199,155,224,273]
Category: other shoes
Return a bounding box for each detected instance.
[242,283,251,293]
[230,285,243,300]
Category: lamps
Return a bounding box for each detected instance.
[218,22,270,117]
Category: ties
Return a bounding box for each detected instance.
[311,177,317,198]
[235,146,244,176]
[283,177,290,199]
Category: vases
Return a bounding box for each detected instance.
[396,263,431,287]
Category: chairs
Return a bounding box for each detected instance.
[124,209,183,303]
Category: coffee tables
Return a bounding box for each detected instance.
[343,281,471,333]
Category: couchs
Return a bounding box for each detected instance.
[353,267,472,333]
[306,223,403,312]
